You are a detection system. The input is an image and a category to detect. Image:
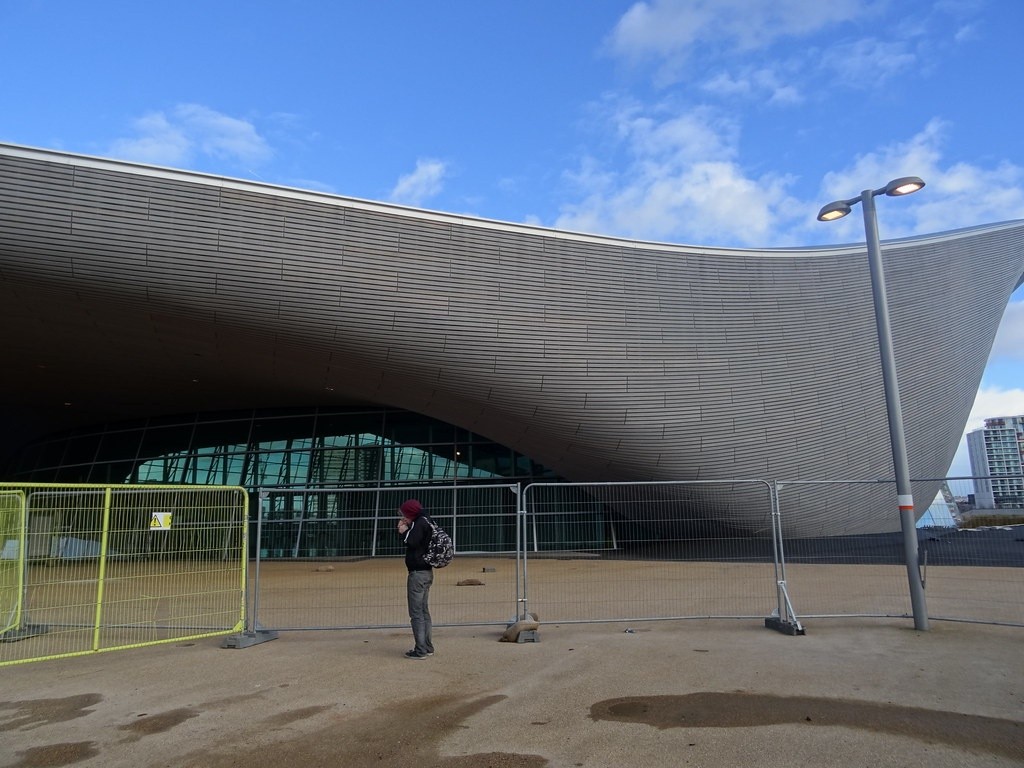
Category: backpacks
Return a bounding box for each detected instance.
[421,517,455,568]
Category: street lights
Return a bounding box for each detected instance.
[816,175,929,634]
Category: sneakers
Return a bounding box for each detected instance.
[405,647,434,660]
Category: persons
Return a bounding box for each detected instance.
[398,499,434,659]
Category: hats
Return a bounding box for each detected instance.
[398,500,422,522]
